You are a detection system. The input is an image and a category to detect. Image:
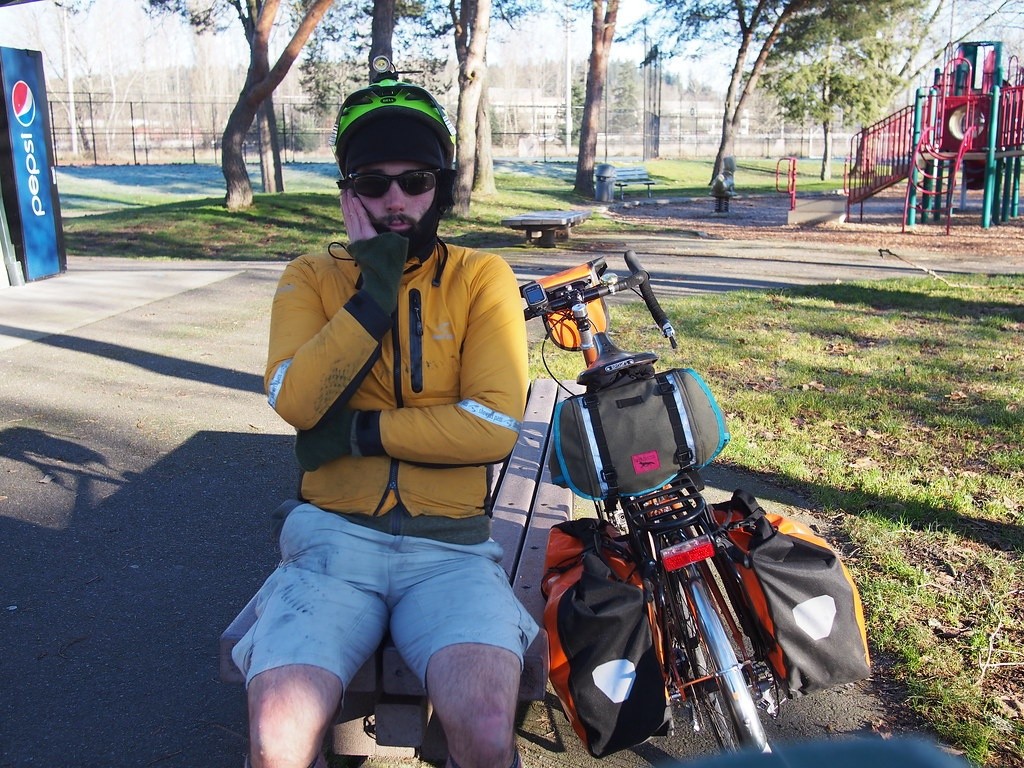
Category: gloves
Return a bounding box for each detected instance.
[295,409,389,473]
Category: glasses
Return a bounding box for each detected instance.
[348,169,439,198]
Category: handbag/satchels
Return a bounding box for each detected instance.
[548,367,730,512]
[707,489,871,700]
[540,516,674,758]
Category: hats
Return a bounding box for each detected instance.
[345,115,445,178]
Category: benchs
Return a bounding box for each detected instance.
[220,380,587,757]
[615,167,655,199]
[501,210,591,241]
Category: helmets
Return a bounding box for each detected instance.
[335,79,454,179]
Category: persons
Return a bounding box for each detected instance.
[232,79,539,768]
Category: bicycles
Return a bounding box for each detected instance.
[517,250,791,756]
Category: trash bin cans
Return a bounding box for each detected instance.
[594,164,617,203]
